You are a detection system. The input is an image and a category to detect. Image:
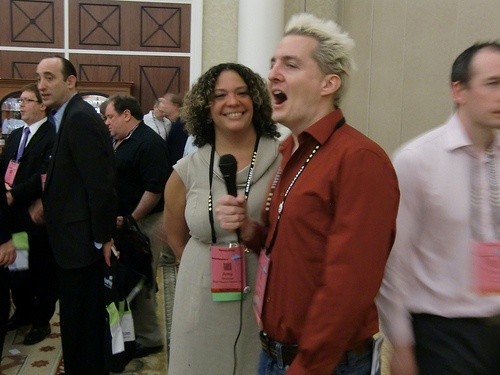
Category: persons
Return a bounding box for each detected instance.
[0,84,56,346]
[29,56,117,375]
[163,64,285,375]
[211,14,400,375]
[94,92,201,359]
[373,39,500,375]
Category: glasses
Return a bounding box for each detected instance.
[18,98,38,103]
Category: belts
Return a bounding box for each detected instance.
[260,330,369,363]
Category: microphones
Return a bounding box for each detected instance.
[218,154,242,243]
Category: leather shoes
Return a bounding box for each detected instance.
[7,320,25,331]
[23,326,50,345]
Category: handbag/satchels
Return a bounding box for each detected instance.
[104,215,153,292]
[105,263,136,355]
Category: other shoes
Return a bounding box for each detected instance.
[160,253,180,266]
[135,346,162,359]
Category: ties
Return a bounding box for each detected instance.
[17,127,30,162]
